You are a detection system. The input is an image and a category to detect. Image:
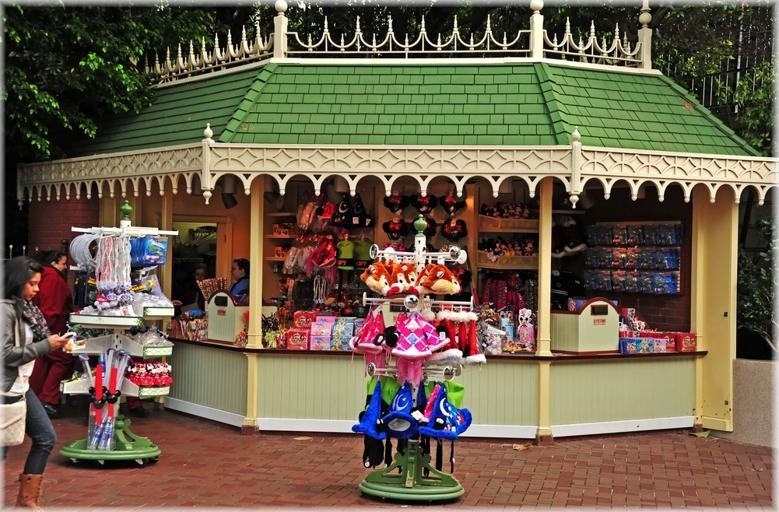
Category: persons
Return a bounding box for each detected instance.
[32,251,73,416]
[0,256,67,512]
[226,258,249,303]
[172,262,206,311]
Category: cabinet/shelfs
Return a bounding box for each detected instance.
[478,214,539,268]
[59,199,180,467]
[263,211,297,261]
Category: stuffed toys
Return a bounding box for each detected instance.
[350,260,488,473]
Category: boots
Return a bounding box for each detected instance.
[16,473,43,510]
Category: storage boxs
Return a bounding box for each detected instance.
[550,296,622,357]
[206,288,278,344]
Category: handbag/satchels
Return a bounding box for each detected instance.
[0,392,27,448]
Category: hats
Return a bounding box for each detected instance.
[348,310,486,477]
[284,193,367,309]
